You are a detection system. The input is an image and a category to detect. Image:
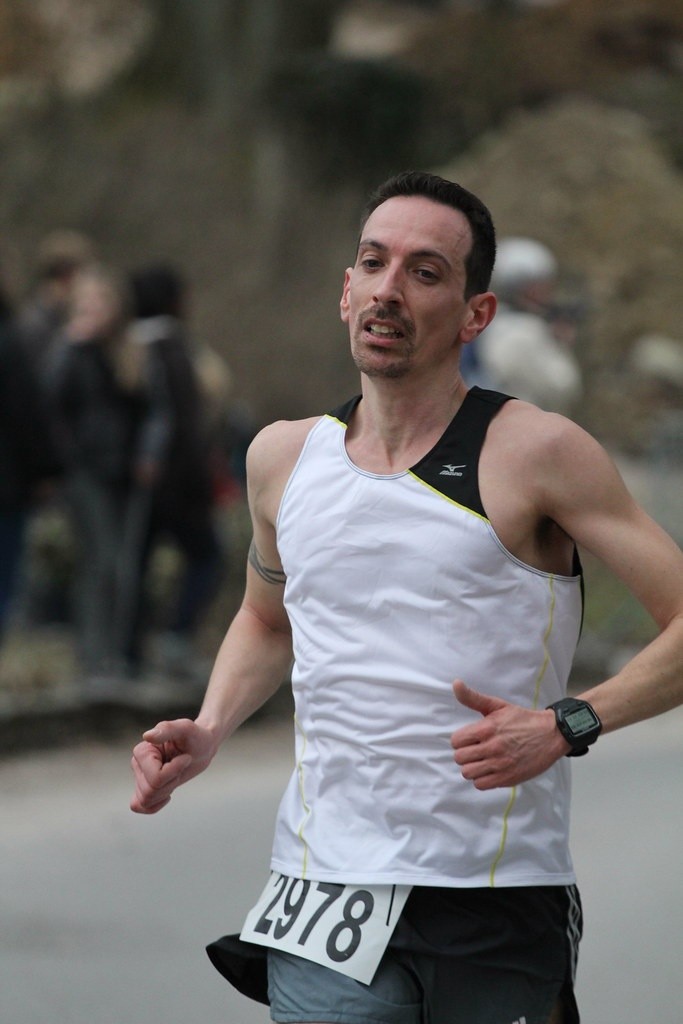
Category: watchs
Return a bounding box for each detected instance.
[545,696,602,759]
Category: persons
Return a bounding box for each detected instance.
[130,167,683,1024]
[0,230,592,691]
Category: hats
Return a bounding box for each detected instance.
[489,233,555,295]
[128,265,179,317]
